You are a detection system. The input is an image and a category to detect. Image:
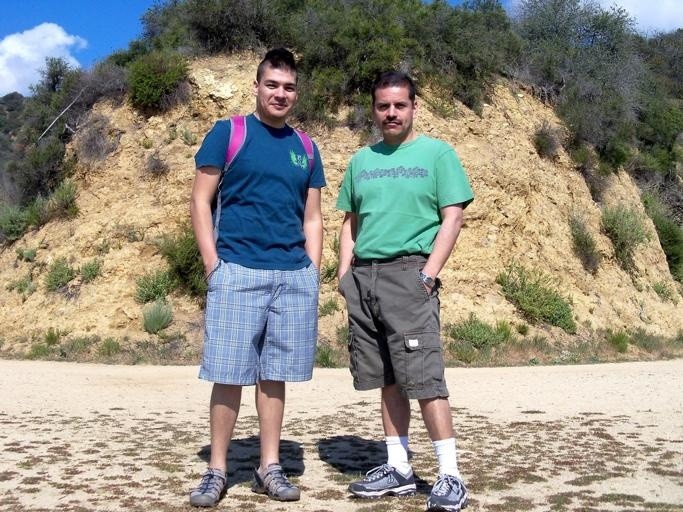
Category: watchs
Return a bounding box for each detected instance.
[419,271,435,288]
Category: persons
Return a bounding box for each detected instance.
[186,49,326,507]
[336,72,474,511]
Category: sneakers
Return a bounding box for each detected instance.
[348,463,417,500]
[190,466,228,507]
[251,463,300,502]
[424,473,469,512]
